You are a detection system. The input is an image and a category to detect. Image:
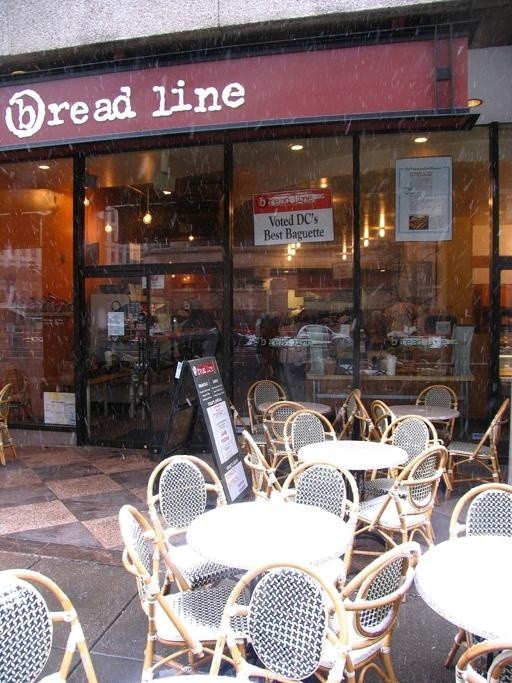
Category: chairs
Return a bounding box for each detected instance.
[119,321,511,603]
[119,505,252,683]
[209,562,355,683]
[146,454,247,597]
[1,568,97,682]
[314,541,422,683]
[413,482,511,683]
[278,458,360,591]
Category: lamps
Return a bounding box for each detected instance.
[283,173,398,264]
[81,161,177,234]
